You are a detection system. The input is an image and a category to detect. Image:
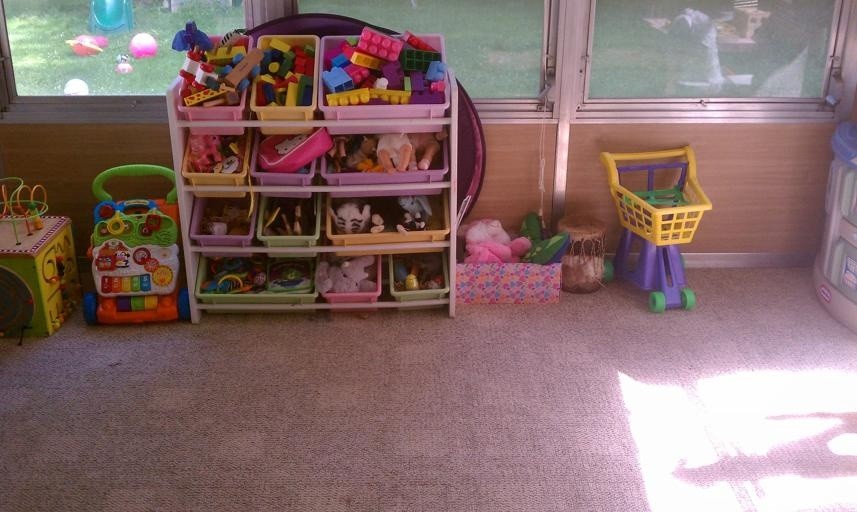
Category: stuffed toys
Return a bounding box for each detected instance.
[316,255,376,294]
[457,212,568,264]
[330,195,430,234]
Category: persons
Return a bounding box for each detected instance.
[328,128,447,174]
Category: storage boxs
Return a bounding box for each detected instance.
[457,256,564,306]
